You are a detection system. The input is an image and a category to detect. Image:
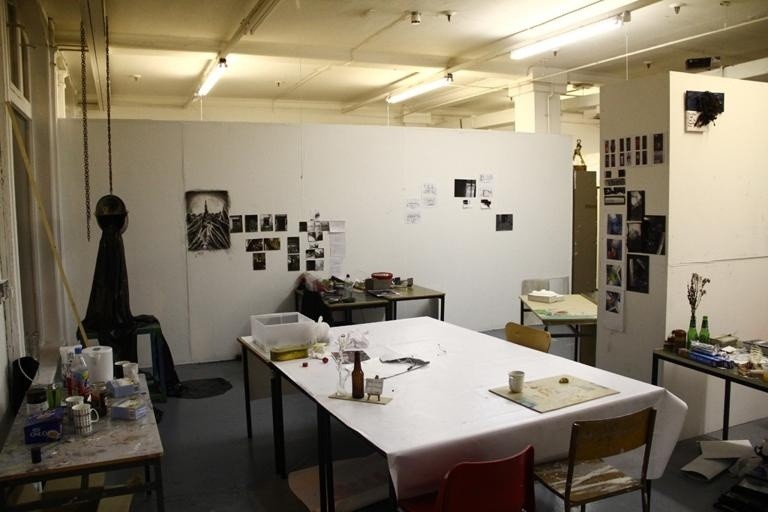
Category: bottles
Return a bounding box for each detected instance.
[327,279,333,290]
[26,388,49,416]
[350,351,366,400]
[81,345,114,383]
[342,273,353,299]
[69,346,90,397]
[699,315,710,344]
[90,382,108,418]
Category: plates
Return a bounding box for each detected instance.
[371,272,393,280]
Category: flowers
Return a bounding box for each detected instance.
[686,272,710,316]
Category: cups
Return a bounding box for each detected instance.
[507,370,525,393]
[761,364,768,381]
[115,360,139,382]
[71,404,99,434]
[65,395,84,417]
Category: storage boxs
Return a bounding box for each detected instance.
[251,311,316,353]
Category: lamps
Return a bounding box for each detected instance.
[385,73,455,104]
[198,58,230,96]
[241,1,280,35]
[510,11,631,61]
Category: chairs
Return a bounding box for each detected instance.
[399,444,536,512]
[534,406,657,512]
[505,323,551,352]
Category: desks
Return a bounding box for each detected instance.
[295,287,393,323]
[518,293,598,361]
[0,373,165,512]
[651,340,768,441]
[237,315,689,512]
[77,314,165,404]
[354,282,446,321]
